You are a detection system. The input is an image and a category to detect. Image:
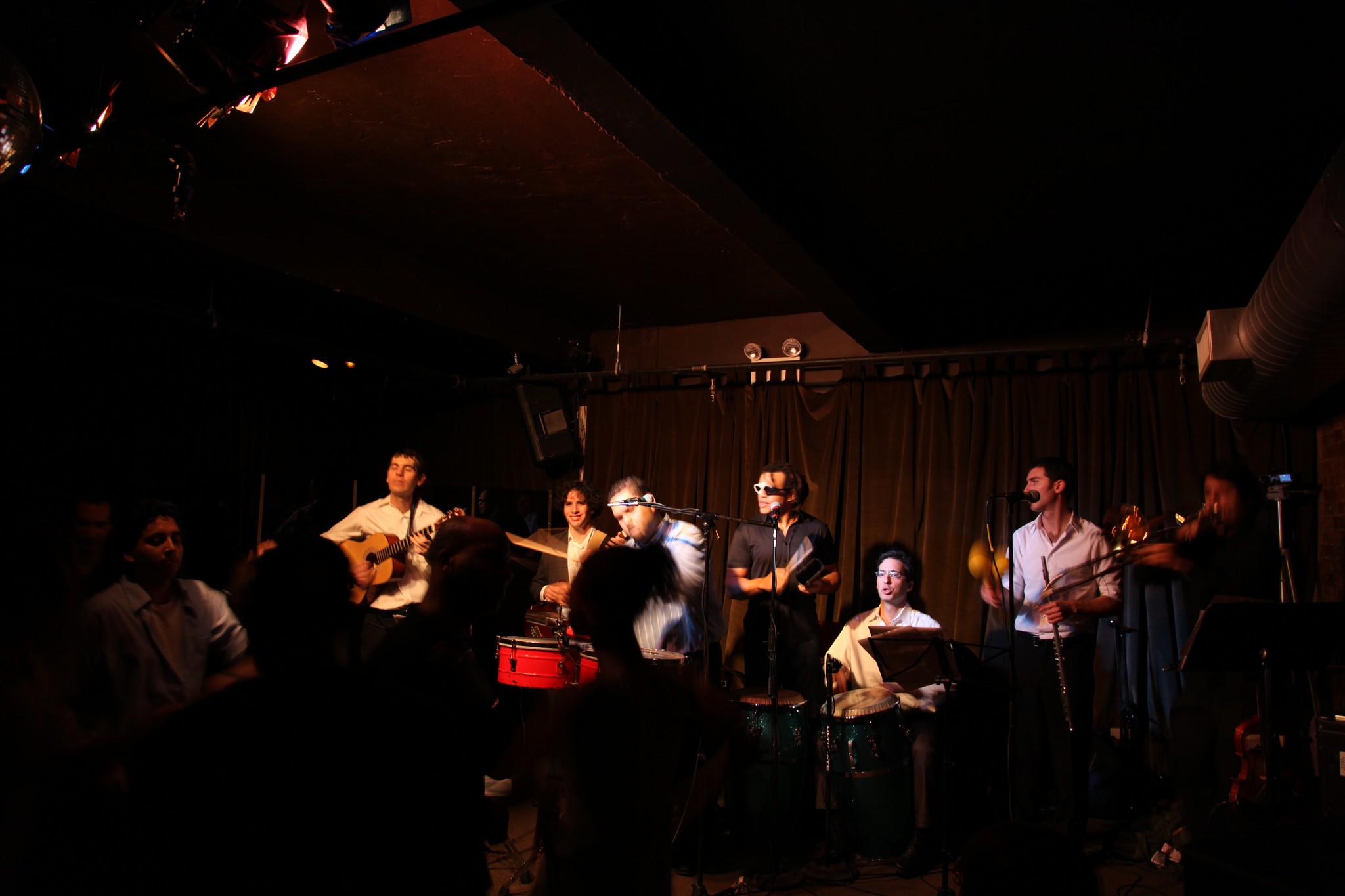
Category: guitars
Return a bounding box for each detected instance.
[338,506,466,606]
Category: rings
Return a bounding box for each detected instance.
[547,598,550,601]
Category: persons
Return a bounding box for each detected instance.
[0,449,724,895]
[981,452,1124,831]
[824,544,961,872]
[1130,470,1316,817]
[725,462,840,693]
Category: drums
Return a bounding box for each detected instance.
[820,687,906,773]
[729,689,813,817]
[498,633,591,689]
[576,644,691,691]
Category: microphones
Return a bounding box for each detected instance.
[826,653,840,674]
[991,490,1040,503]
[771,502,781,520]
[610,494,654,506]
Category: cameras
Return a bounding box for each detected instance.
[1259,473,1293,487]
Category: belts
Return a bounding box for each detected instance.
[1014,629,1071,650]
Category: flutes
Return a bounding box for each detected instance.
[1042,556,1074,733]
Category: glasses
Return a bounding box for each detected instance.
[753,484,791,497]
[875,570,905,580]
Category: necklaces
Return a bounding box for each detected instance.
[779,517,795,530]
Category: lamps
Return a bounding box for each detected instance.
[745,338,802,385]
[0,0,412,182]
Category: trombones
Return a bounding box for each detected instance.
[1032,516,1213,608]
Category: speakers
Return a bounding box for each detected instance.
[515,384,576,466]
